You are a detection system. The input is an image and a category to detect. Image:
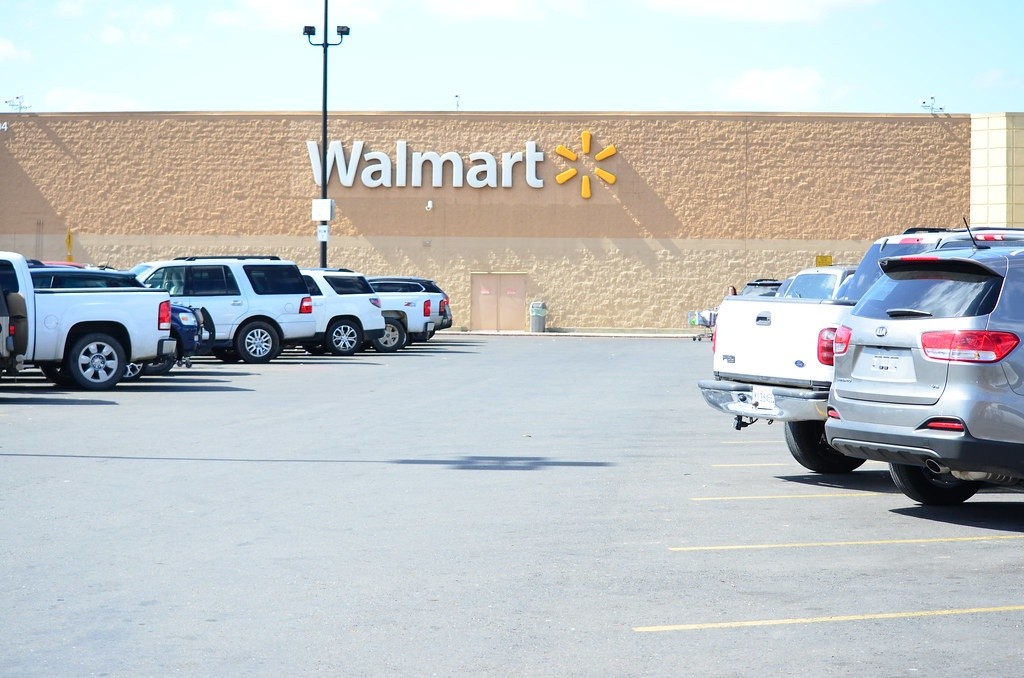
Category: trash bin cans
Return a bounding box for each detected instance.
[529,301,547,333]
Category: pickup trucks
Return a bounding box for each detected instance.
[697,226,1023,475]
[0,251,178,394]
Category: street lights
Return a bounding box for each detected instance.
[302,0,350,270]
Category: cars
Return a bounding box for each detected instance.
[729,268,875,304]
[823,245,1024,506]
[0,258,206,375]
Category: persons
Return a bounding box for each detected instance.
[728,285,738,296]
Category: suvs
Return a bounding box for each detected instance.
[122,255,317,365]
[354,276,453,354]
[224,268,386,357]
